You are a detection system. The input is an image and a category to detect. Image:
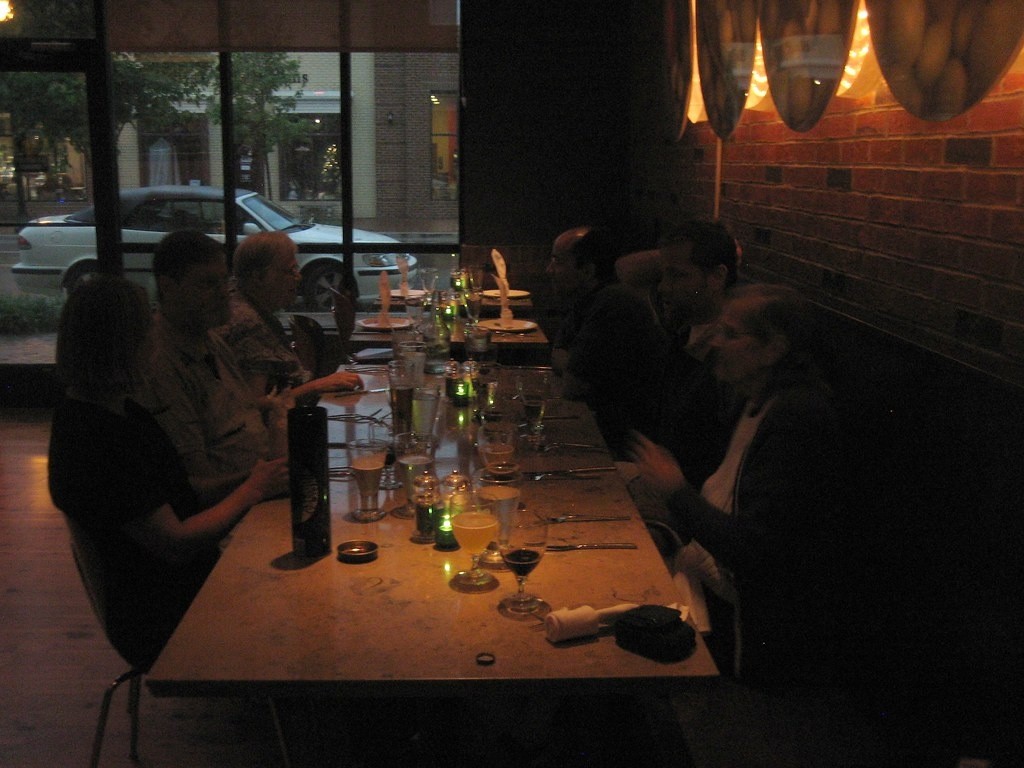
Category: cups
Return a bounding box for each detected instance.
[419,267,439,294]
[476,422,517,466]
[517,374,556,442]
[409,384,443,447]
[385,360,417,432]
[462,326,490,363]
[463,291,482,326]
[471,363,503,422]
[468,264,487,293]
[392,433,437,518]
[473,468,523,572]
[347,440,388,526]
[397,341,428,381]
[404,292,428,331]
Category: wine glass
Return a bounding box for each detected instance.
[498,508,552,620]
[448,490,500,594]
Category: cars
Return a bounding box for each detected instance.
[7,185,418,314]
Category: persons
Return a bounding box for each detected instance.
[546,218,844,682]
[47,277,289,674]
[208,231,364,427]
[129,230,295,510]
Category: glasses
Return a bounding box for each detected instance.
[197,275,239,290]
[712,323,757,342]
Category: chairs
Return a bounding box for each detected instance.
[63,513,292,768]
[331,306,356,357]
[289,314,334,378]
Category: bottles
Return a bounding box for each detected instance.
[424,292,451,372]
[446,267,471,326]
[410,469,475,551]
[284,394,332,561]
[443,358,484,397]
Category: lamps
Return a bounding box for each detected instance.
[387,111,393,123]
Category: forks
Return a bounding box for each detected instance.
[545,543,638,553]
[521,472,608,482]
[535,510,631,523]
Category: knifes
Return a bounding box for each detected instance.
[334,387,392,395]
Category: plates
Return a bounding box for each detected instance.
[388,289,428,300]
[355,316,411,329]
[480,289,530,298]
[478,318,539,332]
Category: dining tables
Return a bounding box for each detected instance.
[143,362,718,768]
[350,289,550,348]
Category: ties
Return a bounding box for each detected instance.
[679,322,693,347]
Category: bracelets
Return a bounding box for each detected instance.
[245,483,262,500]
